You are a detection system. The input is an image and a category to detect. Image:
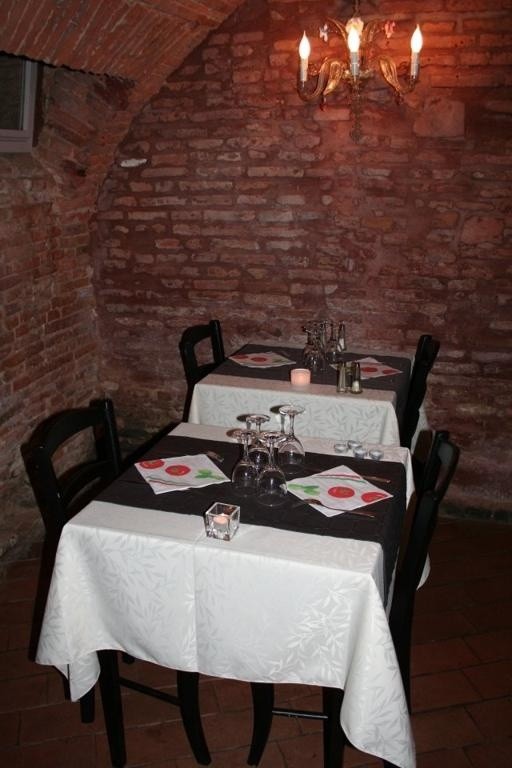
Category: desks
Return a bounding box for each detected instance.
[39,422,424,767]
[188,337,431,456]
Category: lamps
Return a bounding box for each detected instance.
[297,0,423,142]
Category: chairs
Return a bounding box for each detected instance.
[383,427,458,717]
[180,320,224,421]
[20,393,123,664]
[398,333,441,453]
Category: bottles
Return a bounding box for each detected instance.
[336,359,362,394]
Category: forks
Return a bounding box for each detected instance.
[297,499,378,518]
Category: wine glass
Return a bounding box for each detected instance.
[300,321,341,374]
[229,406,305,505]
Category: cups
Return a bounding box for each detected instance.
[205,502,241,541]
